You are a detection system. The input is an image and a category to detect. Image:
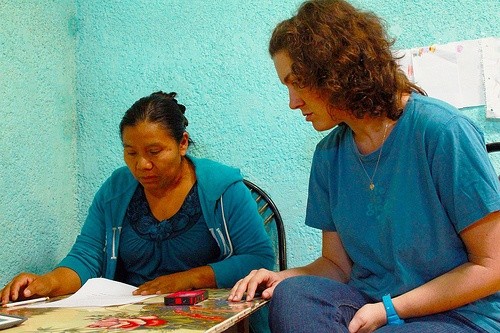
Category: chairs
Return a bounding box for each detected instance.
[238,180,288,289]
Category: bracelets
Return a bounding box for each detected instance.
[383,293,404,327]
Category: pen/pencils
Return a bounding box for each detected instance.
[1,296,50,308]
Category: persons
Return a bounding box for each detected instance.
[0,91,279,333]
[228,0,500,333]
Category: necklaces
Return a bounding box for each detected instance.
[352,119,389,190]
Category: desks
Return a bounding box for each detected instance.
[0,287,273,332]
